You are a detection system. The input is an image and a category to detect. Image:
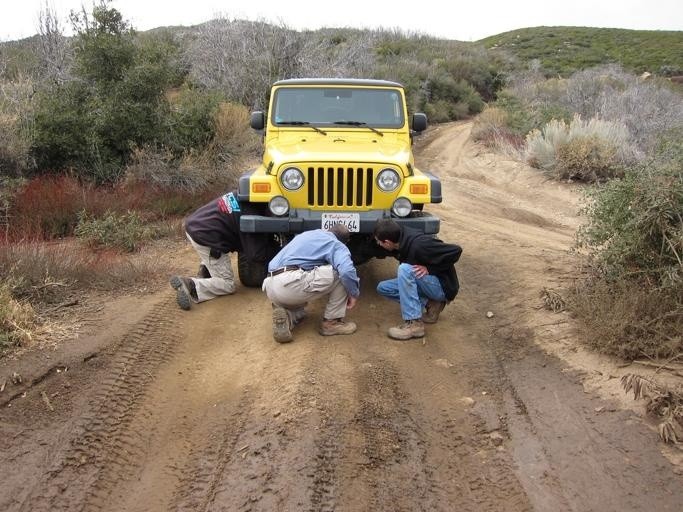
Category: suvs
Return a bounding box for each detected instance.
[224,71,444,292]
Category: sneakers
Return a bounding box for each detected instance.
[386,320,426,341]
[318,318,357,337]
[422,299,447,323]
[272,307,294,343]
[169,275,194,311]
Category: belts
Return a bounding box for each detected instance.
[266,265,298,277]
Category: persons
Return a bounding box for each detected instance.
[261,223,361,344]
[372,218,463,340]
[170,190,272,311]
